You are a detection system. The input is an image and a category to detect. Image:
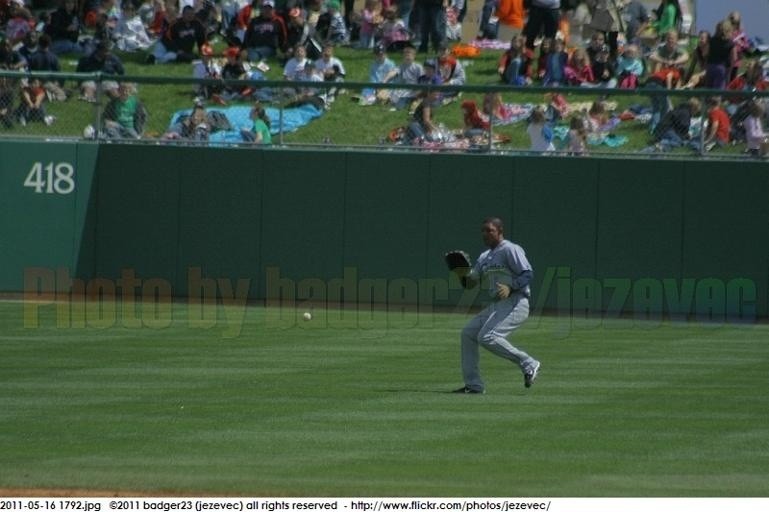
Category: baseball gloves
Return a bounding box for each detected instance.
[444,250,472,278]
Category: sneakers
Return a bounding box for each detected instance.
[451,386,486,394]
[524,360,540,388]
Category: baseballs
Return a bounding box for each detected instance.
[303,313,311,320]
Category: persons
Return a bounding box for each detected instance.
[443,218,542,393]
